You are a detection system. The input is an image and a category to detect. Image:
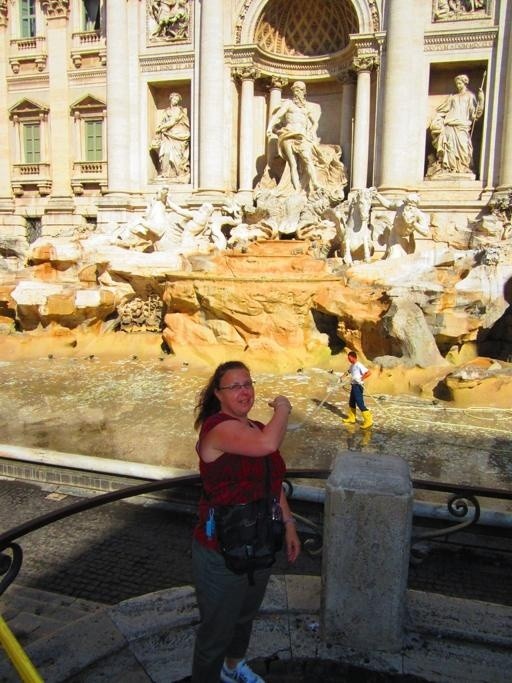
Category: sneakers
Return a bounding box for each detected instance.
[221,658,267,682]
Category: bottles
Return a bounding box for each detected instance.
[270,496,283,522]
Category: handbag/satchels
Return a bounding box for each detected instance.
[214,493,283,574]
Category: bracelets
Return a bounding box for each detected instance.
[275,402,291,412]
[283,516,296,528]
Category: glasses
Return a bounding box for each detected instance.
[218,382,256,391]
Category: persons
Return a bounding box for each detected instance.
[186,361,301,683]
[146,185,430,267]
[337,352,373,429]
[427,74,485,174]
[267,81,322,189]
[155,92,191,178]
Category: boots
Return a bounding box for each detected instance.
[342,407,357,423]
[360,409,373,429]
